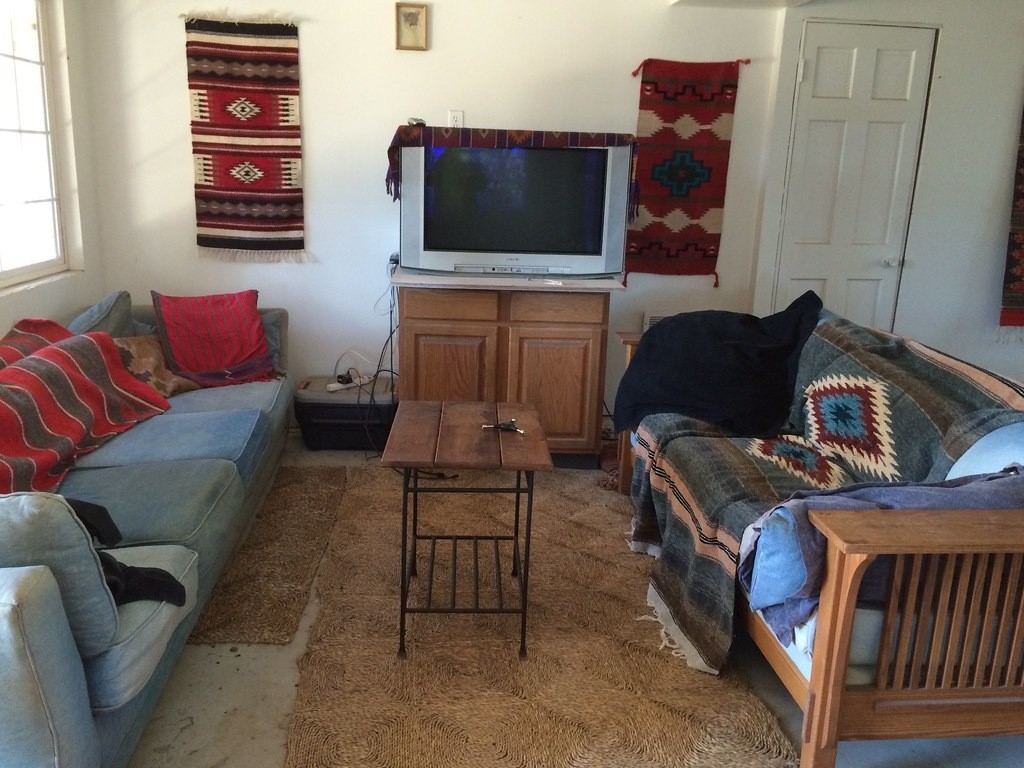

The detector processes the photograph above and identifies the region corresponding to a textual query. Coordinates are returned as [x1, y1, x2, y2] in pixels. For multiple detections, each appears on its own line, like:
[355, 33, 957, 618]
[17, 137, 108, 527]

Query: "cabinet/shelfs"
[391, 264, 626, 466]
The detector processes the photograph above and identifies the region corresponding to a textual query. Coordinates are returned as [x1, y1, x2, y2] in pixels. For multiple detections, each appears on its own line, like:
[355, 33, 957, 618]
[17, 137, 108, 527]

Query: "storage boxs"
[294, 374, 399, 453]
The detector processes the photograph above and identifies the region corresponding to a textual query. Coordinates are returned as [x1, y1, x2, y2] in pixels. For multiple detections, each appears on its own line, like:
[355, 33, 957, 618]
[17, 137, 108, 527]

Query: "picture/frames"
[395, 2, 427, 51]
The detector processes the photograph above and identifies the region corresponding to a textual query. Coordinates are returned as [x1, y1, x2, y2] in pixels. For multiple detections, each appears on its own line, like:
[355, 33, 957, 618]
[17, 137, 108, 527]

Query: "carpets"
[184, 465, 348, 646]
[281, 464, 804, 768]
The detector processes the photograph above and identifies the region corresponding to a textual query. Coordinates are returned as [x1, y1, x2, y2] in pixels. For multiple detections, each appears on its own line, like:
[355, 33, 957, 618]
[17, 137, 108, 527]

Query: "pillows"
[109, 335, 202, 397]
[151, 289, 277, 389]
[66, 290, 157, 340]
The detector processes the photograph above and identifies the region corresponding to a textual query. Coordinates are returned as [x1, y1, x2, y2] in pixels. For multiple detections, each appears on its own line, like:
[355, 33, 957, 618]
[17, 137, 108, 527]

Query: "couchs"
[616, 305, 1024, 767]
[0, 299, 289, 767]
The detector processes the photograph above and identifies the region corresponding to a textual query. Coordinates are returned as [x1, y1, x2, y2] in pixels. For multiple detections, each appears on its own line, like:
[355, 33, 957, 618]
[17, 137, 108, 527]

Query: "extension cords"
[326, 375, 374, 391]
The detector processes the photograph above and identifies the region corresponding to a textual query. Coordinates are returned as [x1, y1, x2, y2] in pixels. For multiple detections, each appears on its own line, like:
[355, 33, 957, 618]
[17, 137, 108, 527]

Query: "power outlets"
[447, 109, 465, 128]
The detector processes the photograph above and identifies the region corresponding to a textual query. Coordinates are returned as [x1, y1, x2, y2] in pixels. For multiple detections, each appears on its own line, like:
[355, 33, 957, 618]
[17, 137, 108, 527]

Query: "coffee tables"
[381, 399, 553, 664]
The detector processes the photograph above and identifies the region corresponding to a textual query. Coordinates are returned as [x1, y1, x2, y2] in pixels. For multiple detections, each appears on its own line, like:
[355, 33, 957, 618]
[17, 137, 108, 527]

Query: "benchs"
[0, 491, 119, 659]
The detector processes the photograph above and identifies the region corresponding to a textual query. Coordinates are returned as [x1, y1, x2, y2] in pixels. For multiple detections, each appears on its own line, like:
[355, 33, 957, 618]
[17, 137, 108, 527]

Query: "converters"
[337, 373, 353, 384]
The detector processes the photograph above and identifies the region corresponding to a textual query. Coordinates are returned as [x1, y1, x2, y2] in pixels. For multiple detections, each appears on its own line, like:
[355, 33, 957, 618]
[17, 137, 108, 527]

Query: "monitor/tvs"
[398, 125, 632, 276]
